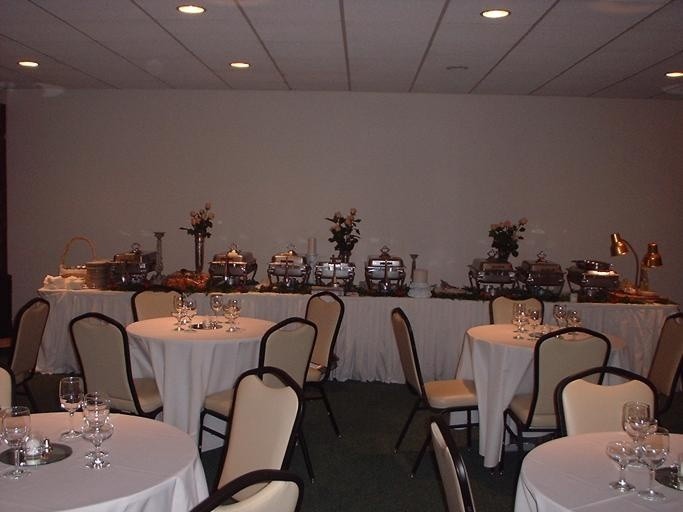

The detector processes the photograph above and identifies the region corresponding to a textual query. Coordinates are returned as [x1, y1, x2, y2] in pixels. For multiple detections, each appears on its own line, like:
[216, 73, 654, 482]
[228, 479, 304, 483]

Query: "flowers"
[324, 207, 361, 257]
[488, 218, 528, 261]
[180, 202, 215, 238]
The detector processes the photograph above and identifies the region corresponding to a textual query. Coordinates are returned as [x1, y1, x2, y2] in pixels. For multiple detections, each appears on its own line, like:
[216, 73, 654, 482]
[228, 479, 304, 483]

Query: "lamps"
[610, 231, 662, 298]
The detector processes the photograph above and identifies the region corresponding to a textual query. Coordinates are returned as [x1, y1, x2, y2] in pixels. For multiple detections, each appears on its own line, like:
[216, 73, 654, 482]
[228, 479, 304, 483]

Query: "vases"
[195, 234, 205, 274]
[500, 251, 508, 261]
[342, 255, 349, 263]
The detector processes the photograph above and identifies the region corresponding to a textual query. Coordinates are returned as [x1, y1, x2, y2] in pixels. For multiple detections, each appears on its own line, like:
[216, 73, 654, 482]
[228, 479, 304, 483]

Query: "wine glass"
[511, 303, 580, 342]
[606, 401, 670, 500]
[1, 378, 117, 481]
[172, 293, 240, 333]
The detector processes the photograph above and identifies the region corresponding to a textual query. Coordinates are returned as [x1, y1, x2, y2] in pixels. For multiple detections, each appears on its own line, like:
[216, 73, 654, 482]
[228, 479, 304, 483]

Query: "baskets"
[58, 236, 97, 284]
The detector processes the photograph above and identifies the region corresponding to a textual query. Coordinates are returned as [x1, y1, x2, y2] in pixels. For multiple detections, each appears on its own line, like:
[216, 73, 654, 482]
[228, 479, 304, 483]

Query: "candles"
[227, 249, 237, 257]
[414, 269, 427, 284]
[309, 237, 316, 254]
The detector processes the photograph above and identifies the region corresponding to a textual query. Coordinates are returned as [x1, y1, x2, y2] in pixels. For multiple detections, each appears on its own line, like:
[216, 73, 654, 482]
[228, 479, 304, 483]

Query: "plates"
[84, 261, 110, 289]
